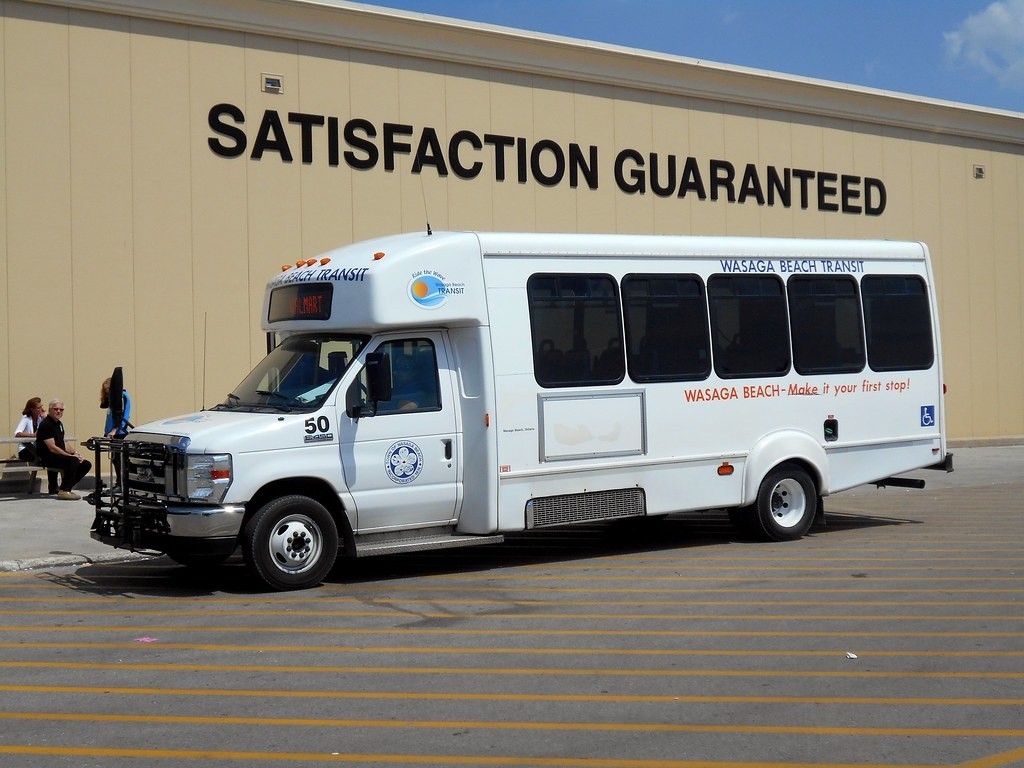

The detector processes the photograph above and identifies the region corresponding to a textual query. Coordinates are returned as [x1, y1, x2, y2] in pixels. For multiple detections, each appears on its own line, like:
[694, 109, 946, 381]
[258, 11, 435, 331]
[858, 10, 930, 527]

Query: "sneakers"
[58, 490, 81, 500]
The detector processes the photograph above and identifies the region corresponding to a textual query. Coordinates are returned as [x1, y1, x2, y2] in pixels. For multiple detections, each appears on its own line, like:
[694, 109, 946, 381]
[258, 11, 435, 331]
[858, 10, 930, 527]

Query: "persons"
[15, 397, 60, 494]
[36, 400, 92, 500]
[100, 377, 131, 493]
[380, 354, 423, 411]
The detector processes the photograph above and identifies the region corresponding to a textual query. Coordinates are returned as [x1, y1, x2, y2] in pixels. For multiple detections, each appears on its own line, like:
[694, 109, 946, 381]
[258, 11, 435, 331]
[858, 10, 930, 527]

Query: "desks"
[0, 437, 78, 495]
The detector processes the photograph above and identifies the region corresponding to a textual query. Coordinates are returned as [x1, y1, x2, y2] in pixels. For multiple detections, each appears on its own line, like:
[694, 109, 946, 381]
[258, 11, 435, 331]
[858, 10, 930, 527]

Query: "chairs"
[723, 332, 765, 375]
[633, 336, 675, 375]
[533, 338, 576, 389]
[593, 338, 632, 383]
[415, 350, 435, 388]
[266, 343, 325, 406]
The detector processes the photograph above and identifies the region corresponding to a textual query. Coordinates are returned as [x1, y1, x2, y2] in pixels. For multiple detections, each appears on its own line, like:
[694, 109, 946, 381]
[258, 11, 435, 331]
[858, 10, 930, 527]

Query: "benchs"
[0, 458, 26, 464]
[0, 466, 62, 473]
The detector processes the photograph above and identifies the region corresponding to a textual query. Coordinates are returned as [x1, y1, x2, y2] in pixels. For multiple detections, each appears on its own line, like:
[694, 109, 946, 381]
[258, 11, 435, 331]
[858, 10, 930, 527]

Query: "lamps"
[972, 164, 985, 179]
[261, 72, 284, 94]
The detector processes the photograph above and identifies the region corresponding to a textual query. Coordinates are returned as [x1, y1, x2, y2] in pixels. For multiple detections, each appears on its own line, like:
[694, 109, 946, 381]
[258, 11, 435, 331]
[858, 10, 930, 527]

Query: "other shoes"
[106, 485, 122, 494]
[49, 493, 59, 497]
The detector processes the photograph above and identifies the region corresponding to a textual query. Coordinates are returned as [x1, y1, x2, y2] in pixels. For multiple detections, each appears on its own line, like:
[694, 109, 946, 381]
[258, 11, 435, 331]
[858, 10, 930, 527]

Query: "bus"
[81, 231, 955, 593]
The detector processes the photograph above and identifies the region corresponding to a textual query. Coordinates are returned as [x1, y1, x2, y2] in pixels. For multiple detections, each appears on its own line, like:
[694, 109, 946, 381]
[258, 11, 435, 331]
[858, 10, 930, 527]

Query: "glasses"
[53, 408, 64, 411]
[396, 364, 414, 372]
